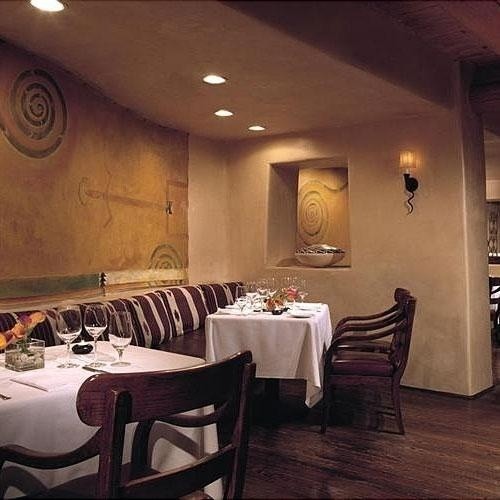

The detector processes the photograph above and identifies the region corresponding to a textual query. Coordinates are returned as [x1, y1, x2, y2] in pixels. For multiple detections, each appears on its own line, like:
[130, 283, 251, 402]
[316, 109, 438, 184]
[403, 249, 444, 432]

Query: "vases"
[4, 336, 46, 371]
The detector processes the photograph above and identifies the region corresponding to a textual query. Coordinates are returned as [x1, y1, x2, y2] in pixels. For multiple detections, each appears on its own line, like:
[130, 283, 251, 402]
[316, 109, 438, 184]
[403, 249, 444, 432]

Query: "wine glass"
[83, 304, 107, 368]
[108, 311, 132, 367]
[56, 304, 82, 369]
[235, 276, 309, 318]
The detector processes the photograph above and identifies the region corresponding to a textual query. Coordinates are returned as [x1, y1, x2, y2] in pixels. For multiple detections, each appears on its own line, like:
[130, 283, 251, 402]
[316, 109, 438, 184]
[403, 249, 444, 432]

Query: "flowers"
[16, 313, 34, 355]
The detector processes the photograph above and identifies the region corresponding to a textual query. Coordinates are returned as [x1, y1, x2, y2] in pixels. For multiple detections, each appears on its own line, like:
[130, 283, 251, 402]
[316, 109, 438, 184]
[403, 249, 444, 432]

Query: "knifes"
[82, 366, 111, 374]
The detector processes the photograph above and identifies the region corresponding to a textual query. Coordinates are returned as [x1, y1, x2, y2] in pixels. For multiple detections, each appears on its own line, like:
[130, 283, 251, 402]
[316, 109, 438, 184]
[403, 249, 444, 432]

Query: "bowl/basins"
[294, 252, 345, 267]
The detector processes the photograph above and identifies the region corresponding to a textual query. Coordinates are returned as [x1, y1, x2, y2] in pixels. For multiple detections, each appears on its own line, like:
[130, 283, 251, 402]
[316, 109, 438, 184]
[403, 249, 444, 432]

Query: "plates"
[220, 305, 250, 315]
[289, 310, 312, 317]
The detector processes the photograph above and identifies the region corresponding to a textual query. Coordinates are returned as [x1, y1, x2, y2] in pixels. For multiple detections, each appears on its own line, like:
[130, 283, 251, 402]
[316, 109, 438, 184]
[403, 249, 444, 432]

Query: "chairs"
[322, 284, 417, 437]
[0, 349, 257, 500]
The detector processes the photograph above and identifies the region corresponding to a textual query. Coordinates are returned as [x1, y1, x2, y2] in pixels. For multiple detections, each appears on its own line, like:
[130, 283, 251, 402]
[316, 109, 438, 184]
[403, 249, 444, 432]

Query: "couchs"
[0, 276, 246, 362]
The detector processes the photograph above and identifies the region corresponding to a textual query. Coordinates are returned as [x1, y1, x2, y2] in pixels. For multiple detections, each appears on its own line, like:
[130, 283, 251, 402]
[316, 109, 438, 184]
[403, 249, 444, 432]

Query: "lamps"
[399, 149, 418, 193]
